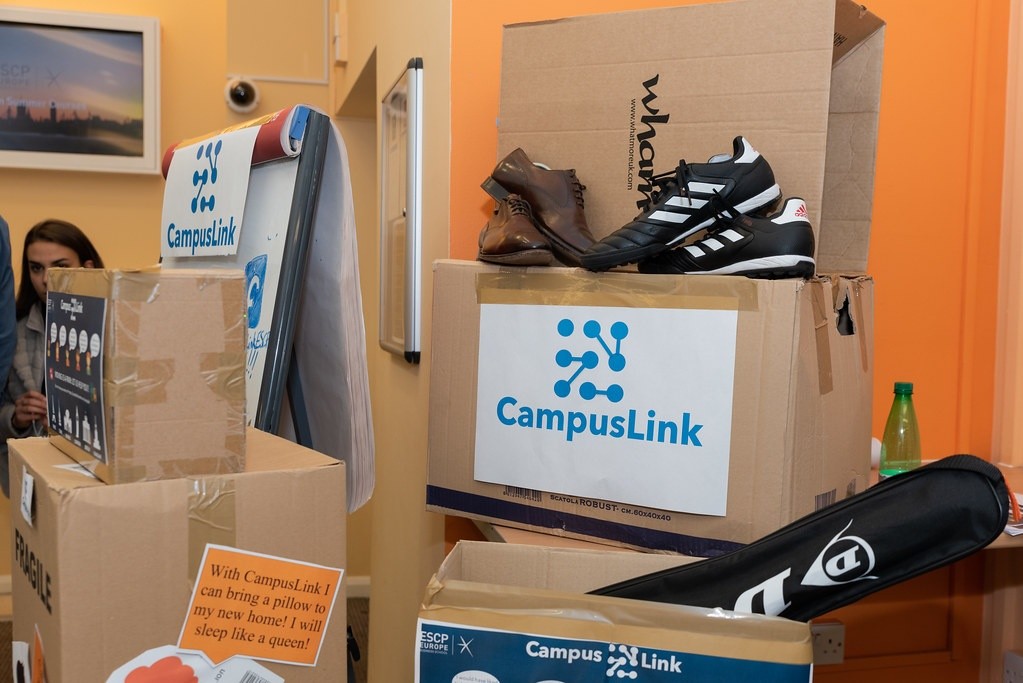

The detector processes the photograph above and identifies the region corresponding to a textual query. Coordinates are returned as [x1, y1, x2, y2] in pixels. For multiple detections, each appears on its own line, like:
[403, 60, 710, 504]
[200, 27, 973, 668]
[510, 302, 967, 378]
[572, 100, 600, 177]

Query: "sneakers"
[579, 135, 783, 269]
[638, 196, 815, 279]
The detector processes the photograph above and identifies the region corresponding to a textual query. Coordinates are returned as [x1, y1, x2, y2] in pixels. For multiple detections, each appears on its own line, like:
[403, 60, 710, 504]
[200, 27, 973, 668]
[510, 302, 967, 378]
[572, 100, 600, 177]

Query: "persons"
[0, 218, 106, 445]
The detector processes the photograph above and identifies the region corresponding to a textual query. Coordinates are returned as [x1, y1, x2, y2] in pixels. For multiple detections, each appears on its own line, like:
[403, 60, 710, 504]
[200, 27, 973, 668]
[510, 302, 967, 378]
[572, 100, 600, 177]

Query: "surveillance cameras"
[225, 78, 259, 113]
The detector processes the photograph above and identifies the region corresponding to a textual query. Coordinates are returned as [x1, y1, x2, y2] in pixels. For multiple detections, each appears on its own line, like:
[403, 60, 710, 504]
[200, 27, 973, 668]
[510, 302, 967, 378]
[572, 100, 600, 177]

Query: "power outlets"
[812, 622, 848, 667]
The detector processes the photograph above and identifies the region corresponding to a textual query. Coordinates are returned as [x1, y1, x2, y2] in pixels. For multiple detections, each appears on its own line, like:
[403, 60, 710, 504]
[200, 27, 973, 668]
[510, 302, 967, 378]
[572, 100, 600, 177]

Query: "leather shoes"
[476, 148, 598, 269]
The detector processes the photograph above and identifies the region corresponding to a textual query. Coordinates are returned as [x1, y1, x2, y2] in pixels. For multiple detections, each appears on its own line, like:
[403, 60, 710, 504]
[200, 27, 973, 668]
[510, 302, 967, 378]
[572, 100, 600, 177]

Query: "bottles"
[878, 380, 921, 482]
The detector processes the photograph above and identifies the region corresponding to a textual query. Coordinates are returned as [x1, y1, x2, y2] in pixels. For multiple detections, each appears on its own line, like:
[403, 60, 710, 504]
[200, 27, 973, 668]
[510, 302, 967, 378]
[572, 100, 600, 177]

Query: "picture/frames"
[0, 6, 165, 176]
[373, 56, 429, 368]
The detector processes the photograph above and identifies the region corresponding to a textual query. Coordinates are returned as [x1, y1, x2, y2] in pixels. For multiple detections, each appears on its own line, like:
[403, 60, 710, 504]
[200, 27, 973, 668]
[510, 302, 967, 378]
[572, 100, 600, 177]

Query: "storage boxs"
[7, 426, 348, 683]
[414, 533, 816, 683]
[497, 1, 890, 279]
[426, 255, 877, 553]
[45, 267, 249, 475]
